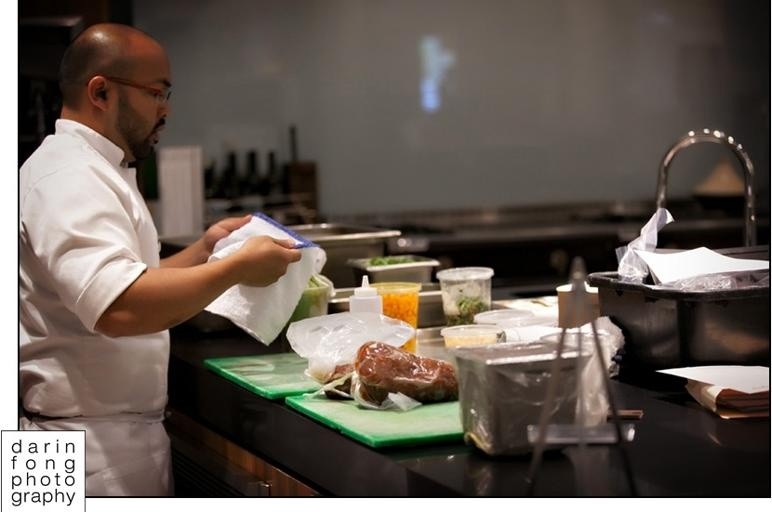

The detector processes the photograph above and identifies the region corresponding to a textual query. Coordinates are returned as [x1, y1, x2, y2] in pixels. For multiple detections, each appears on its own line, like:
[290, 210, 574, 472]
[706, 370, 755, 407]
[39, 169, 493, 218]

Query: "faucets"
[656, 129, 759, 246]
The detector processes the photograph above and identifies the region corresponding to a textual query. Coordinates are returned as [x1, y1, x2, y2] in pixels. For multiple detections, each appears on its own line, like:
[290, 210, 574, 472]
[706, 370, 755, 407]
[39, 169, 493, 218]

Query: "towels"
[198, 214, 329, 347]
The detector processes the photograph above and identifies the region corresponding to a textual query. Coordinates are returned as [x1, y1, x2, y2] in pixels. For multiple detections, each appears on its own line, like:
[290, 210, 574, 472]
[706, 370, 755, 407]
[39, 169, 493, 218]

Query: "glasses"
[86, 74, 170, 103]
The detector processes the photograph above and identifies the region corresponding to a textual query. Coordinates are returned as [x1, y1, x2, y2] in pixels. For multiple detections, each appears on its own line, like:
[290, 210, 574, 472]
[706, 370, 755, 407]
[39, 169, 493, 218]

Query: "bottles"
[349, 275, 383, 320]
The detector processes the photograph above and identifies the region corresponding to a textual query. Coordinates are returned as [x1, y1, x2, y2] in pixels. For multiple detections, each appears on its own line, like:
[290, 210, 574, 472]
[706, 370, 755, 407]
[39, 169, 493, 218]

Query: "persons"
[17, 22, 303, 498]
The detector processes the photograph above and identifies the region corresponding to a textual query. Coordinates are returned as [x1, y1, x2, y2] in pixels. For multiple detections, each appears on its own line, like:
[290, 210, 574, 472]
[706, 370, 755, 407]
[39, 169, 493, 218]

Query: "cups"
[435, 265, 496, 326]
[369, 281, 422, 354]
